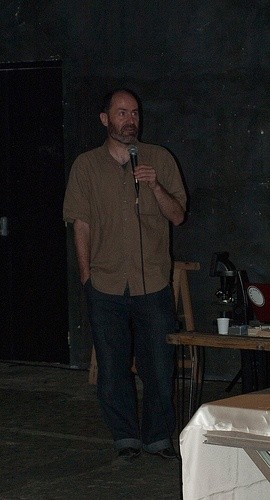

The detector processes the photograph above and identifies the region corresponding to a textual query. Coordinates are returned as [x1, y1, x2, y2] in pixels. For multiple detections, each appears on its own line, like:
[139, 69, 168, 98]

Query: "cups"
[216, 317, 230, 335]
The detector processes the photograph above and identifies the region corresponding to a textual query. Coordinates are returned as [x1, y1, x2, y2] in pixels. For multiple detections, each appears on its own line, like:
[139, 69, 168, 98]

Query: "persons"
[60, 84, 189, 462]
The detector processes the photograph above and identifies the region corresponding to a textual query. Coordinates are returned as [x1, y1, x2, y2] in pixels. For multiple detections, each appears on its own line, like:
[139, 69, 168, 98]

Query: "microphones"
[128, 144, 141, 193]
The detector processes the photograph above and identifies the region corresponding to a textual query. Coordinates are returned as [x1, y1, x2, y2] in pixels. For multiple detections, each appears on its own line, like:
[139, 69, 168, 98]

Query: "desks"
[163, 325, 270, 430]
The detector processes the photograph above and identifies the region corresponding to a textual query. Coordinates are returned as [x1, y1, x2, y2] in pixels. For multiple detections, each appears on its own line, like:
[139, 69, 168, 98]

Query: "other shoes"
[153, 447, 177, 459]
[116, 448, 142, 462]
